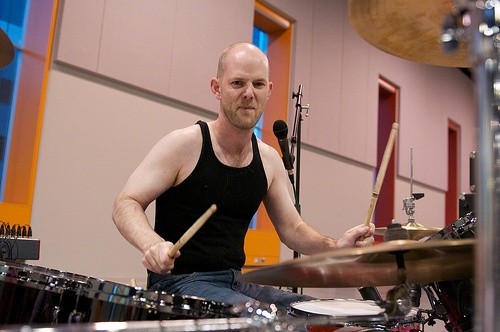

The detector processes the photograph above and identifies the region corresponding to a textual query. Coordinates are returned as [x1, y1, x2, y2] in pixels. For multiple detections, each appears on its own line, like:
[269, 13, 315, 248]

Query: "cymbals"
[237, 239, 477, 287]
[374, 223, 442, 240]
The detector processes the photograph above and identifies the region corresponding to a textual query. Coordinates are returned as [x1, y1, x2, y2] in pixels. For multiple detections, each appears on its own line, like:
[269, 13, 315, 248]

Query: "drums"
[421, 210, 478, 332]
[0, 260, 245, 322]
[287, 297, 389, 332]
[2, 318, 267, 332]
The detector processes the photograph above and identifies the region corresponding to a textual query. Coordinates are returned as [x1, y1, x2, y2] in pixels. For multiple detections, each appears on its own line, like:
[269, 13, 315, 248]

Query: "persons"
[110, 41, 376, 304]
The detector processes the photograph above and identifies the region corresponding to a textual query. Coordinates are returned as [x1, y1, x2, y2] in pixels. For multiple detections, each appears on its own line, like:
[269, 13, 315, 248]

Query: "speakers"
[458, 194, 474, 218]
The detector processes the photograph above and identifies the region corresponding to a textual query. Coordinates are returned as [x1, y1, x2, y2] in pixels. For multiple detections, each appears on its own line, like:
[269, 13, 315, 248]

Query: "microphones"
[272, 119, 295, 185]
